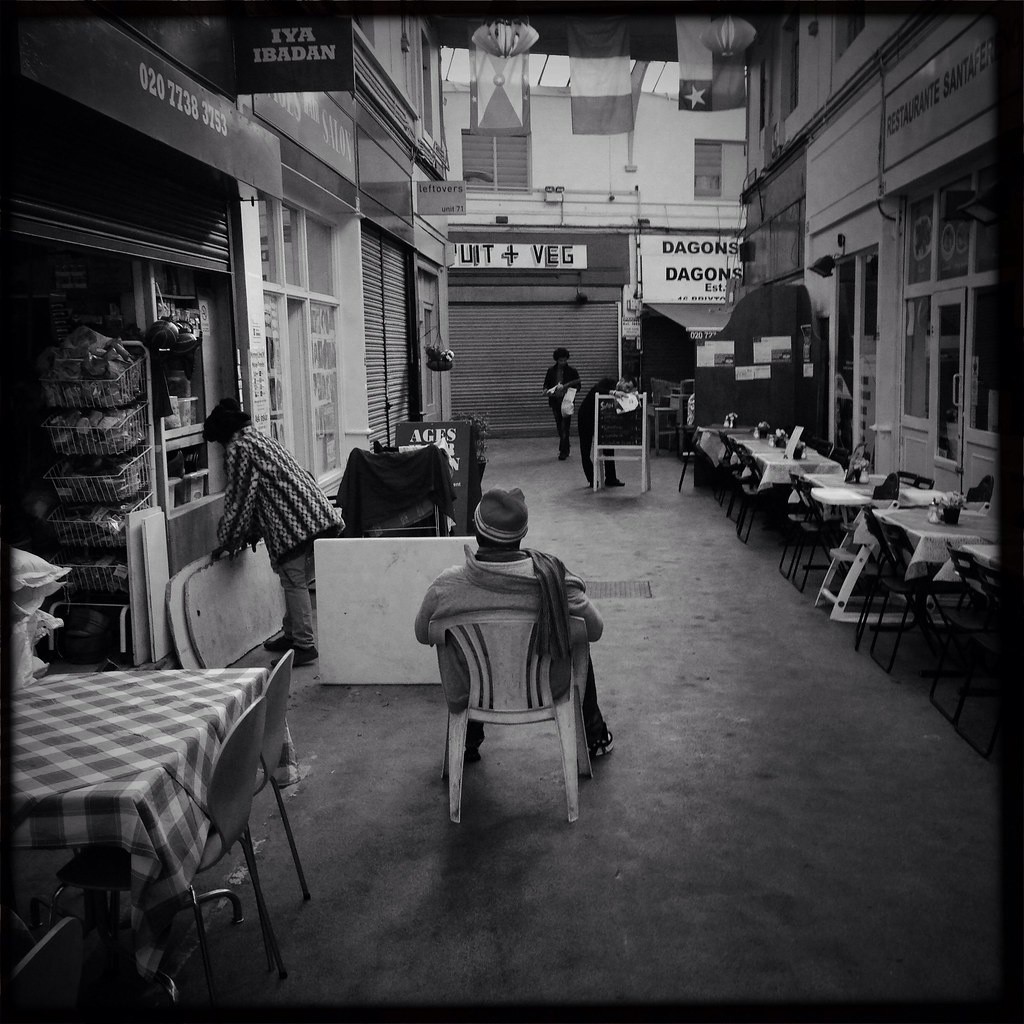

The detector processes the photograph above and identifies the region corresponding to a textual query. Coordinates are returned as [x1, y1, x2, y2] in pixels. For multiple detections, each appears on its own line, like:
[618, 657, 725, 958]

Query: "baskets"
[62, 633, 111, 664]
[40, 356, 150, 594]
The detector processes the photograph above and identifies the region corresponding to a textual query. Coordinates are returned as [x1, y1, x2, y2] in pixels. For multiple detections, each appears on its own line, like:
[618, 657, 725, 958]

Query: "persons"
[543, 347, 581, 461]
[202, 398, 345, 668]
[413, 486, 614, 762]
[578, 375, 639, 488]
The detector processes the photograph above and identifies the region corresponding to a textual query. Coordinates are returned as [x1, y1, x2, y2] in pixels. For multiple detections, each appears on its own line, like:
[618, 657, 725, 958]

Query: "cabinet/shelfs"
[146, 264, 228, 575]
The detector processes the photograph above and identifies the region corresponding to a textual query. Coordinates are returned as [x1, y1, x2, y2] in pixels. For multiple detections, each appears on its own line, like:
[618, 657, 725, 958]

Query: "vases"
[776, 438, 784, 447]
[860, 471, 870, 484]
[729, 420, 736, 428]
[793, 448, 803, 460]
[426, 359, 453, 371]
[943, 508, 961, 525]
[760, 430, 768, 439]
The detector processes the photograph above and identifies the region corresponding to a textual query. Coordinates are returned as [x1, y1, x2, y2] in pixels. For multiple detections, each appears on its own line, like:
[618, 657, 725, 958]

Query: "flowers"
[758, 421, 770, 428]
[853, 459, 869, 471]
[796, 439, 802, 448]
[726, 413, 737, 419]
[935, 490, 968, 512]
[423, 344, 455, 361]
[775, 429, 789, 437]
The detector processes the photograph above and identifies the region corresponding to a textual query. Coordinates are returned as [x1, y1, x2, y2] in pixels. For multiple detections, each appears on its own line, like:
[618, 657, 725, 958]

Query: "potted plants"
[451, 412, 490, 536]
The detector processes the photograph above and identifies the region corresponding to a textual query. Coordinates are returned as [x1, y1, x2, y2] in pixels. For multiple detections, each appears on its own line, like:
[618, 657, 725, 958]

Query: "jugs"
[148, 316, 198, 357]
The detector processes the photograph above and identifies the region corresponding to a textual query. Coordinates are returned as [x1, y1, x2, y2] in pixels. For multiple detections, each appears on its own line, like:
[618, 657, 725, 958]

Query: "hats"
[474, 488, 528, 543]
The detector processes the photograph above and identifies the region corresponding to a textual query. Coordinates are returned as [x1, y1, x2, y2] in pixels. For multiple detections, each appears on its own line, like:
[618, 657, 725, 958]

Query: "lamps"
[808, 254, 835, 278]
[496, 216, 508, 223]
[639, 218, 650, 228]
[545, 185, 565, 203]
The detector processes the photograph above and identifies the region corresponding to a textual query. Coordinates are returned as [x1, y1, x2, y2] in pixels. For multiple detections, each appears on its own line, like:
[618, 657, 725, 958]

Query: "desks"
[933, 545, 998, 580]
[726, 433, 790, 443]
[872, 507, 998, 583]
[693, 425, 754, 440]
[14, 667, 269, 1002]
[751, 452, 845, 492]
[742, 443, 816, 451]
[789, 473, 916, 520]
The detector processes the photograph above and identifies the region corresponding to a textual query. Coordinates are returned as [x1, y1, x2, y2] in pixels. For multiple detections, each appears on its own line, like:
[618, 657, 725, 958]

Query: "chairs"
[427, 612, 588, 825]
[196, 645, 310, 976]
[679, 421, 1000, 724]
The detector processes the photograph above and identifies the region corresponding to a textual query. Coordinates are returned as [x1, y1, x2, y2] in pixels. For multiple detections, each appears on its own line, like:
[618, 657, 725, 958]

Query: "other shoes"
[264, 634, 296, 651]
[589, 730, 614, 756]
[605, 478, 625, 487]
[271, 645, 319, 667]
[464, 750, 481, 763]
[590, 480, 599, 488]
[559, 452, 569, 460]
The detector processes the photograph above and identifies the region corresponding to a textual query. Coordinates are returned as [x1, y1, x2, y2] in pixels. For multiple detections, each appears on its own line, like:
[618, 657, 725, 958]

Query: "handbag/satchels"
[561, 388, 577, 417]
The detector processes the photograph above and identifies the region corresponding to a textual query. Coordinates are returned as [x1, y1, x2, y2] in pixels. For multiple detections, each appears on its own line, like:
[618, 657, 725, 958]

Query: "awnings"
[648, 302, 738, 340]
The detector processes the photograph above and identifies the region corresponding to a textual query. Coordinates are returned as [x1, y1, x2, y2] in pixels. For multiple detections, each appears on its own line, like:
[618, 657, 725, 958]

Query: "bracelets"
[613, 390, 616, 395]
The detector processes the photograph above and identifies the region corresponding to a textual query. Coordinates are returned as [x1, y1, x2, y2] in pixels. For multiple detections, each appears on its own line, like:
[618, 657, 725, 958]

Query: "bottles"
[168, 371, 192, 398]
